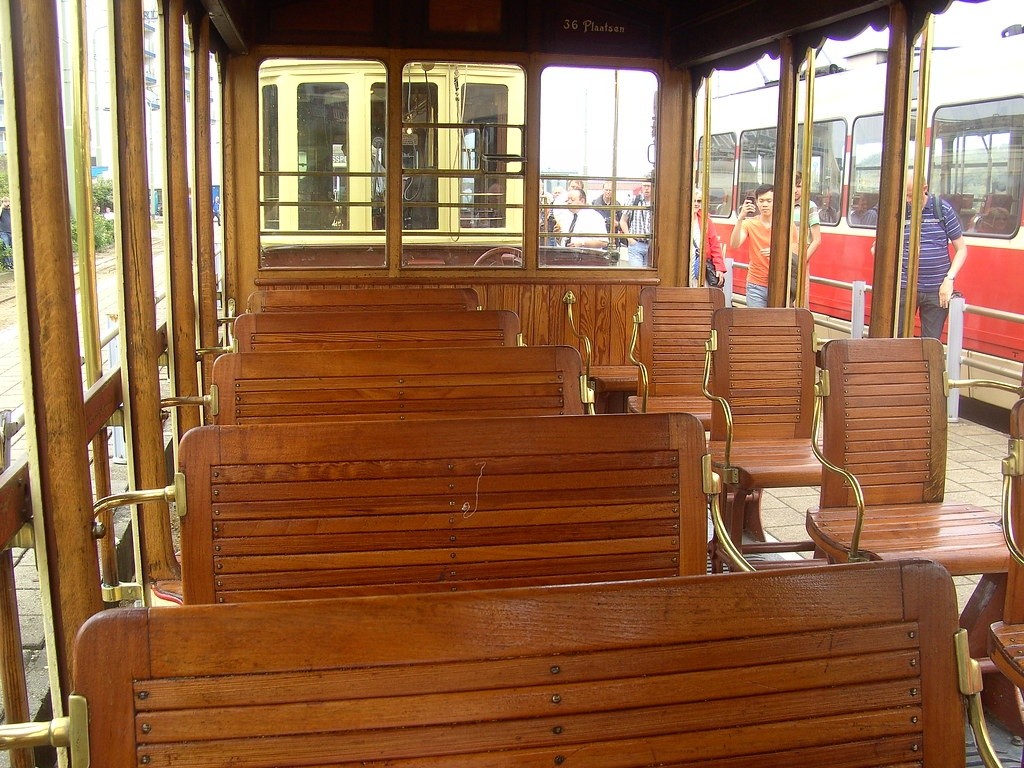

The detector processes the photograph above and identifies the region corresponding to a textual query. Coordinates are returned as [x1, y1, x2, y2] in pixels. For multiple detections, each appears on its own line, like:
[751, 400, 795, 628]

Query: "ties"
[565, 213, 577, 247]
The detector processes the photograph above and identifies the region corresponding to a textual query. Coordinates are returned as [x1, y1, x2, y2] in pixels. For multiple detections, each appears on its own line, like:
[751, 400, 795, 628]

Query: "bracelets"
[945, 274, 954, 280]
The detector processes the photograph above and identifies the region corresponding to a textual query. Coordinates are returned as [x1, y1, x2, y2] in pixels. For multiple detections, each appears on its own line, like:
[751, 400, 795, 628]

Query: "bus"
[239, 41, 527, 269]
[651, 42, 1024, 415]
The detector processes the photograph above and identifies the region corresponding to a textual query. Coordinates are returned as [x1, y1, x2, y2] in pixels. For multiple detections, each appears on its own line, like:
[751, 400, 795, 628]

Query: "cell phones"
[746, 196, 755, 214]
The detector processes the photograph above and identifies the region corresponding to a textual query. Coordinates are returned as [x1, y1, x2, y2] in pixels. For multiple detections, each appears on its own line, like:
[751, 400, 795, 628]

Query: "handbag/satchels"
[620, 222, 630, 247]
[704, 258, 725, 287]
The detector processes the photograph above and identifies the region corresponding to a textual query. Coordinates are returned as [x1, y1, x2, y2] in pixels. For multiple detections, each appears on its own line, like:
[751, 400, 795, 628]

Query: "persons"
[299, 160, 335, 230]
[552, 189, 609, 249]
[0, 196, 14, 272]
[213, 196, 221, 226]
[540, 179, 565, 246]
[790, 171, 821, 307]
[813, 184, 879, 226]
[871, 168, 967, 340]
[567, 180, 584, 192]
[730, 183, 798, 307]
[620, 181, 652, 267]
[692, 187, 728, 292]
[188, 187, 194, 267]
[624, 193, 631, 206]
[592, 182, 622, 250]
[977, 207, 1010, 234]
[468, 183, 504, 227]
[342, 141, 386, 214]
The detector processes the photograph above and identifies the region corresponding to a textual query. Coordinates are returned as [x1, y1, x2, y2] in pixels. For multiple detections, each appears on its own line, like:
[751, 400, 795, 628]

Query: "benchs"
[244, 288, 482, 314]
[68, 556, 1003, 768]
[173, 413, 756, 606]
[207, 346, 594, 425]
[233, 310, 527, 354]
[252, 278, 662, 414]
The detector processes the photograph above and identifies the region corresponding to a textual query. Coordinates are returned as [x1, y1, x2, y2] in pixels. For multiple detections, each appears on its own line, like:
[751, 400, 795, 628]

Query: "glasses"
[693, 199, 702, 203]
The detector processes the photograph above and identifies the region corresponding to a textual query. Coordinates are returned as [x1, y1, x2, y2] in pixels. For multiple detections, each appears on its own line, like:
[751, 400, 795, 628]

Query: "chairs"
[985, 398, 1024, 768]
[700, 307, 824, 574]
[806, 337, 1024, 741]
[625, 286, 726, 431]
